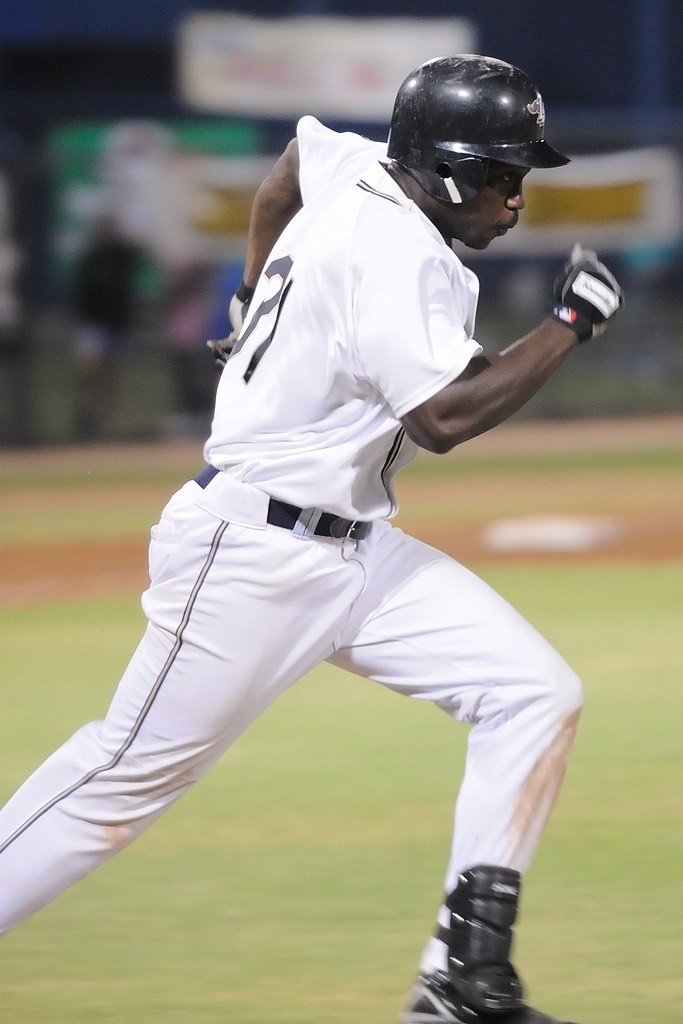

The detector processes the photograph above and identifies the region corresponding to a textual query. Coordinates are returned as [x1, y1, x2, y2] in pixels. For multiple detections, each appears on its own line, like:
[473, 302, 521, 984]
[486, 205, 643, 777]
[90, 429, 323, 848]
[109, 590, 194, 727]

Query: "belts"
[193, 464, 374, 544]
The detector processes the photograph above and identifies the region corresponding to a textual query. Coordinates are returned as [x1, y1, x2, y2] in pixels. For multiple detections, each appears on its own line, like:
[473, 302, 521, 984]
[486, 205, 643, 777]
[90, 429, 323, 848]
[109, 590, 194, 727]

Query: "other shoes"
[407, 966, 572, 1024]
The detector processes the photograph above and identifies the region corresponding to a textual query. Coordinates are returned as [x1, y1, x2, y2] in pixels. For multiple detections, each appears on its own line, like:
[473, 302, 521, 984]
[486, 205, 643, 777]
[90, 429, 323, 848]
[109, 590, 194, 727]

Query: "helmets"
[381, 52, 571, 209]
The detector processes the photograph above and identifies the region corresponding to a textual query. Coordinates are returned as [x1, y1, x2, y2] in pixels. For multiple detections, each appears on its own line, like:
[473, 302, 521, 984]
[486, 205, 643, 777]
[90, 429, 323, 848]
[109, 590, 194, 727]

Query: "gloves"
[206, 280, 255, 365]
[547, 236, 624, 344]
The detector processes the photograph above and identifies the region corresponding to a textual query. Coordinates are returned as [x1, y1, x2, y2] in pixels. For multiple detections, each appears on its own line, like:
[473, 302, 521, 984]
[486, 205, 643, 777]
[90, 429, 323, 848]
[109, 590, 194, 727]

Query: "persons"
[0, 54, 625, 1024]
